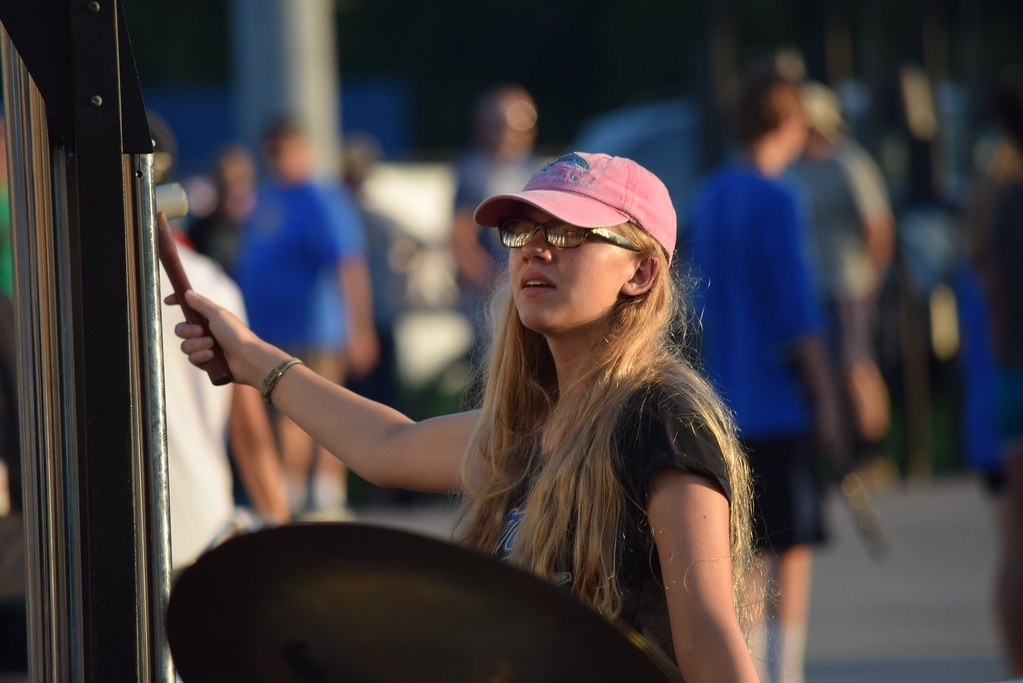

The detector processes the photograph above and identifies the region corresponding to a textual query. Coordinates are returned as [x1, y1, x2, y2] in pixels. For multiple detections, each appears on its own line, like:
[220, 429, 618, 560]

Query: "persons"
[164, 152, 760, 683]
[146, 72, 1023, 683]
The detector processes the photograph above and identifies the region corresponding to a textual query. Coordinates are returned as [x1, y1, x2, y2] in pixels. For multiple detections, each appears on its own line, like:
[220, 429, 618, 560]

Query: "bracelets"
[261, 357, 304, 404]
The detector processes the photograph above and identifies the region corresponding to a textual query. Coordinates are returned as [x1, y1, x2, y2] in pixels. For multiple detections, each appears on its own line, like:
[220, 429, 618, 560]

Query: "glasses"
[497, 216, 643, 252]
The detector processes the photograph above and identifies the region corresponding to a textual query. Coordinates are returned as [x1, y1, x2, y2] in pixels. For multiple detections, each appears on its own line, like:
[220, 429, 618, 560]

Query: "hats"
[474, 152, 677, 270]
